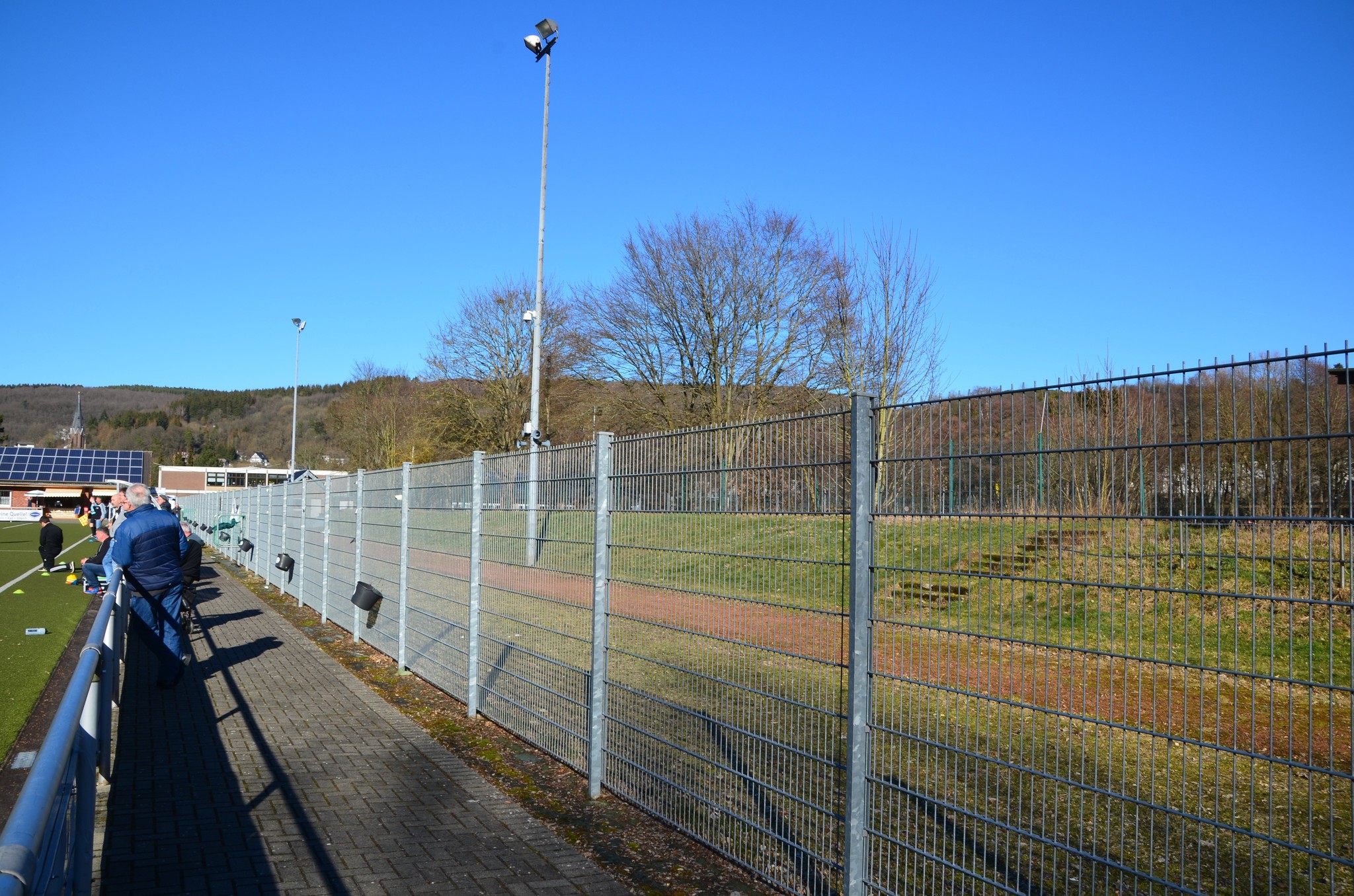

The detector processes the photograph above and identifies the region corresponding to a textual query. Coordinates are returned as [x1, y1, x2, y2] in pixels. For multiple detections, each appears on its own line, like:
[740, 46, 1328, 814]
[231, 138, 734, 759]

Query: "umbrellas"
[24, 490, 45, 507]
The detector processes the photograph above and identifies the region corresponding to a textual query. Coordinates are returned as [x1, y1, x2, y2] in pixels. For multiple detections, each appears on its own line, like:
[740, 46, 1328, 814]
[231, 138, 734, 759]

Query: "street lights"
[290, 317, 308, 482]
[522, 17, 559, 568]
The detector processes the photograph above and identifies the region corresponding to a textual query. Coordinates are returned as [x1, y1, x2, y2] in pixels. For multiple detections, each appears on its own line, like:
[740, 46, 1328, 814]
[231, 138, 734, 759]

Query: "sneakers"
[38, 567, 48, 572]
[84, 587, 102, 594]
[69, 561, 75, 573]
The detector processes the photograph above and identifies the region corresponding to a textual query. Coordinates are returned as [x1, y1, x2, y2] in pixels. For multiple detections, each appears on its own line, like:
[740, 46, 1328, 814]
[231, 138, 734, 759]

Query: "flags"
[78, 513, 88, 526]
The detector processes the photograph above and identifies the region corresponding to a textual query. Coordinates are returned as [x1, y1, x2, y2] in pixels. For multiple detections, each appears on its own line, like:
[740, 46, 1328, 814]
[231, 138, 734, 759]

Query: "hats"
[160, 495, 167, 501]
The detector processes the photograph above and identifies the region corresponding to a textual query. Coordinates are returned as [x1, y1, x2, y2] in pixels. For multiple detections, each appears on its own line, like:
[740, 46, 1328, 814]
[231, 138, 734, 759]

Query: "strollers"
[180, 539, 201, 635]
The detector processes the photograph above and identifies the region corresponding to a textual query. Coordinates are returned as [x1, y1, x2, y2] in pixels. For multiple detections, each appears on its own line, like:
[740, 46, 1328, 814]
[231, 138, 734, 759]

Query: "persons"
[157, 495, 172, 514]
[25, 496, 32, 510]
[111, 482, 192, 691]
[98, 487, 129, 598]
[122, 498, 131, 512]
[172, 503, 183, 521]
[88, 496, 98, 538]
[38, 516, 75, 573]
[92, 496, 106, 542]
[81, 526, 113, 594]
[55, 500, 62, 508]
[182, 521, 204, 590]
[108, 494, 122, 529]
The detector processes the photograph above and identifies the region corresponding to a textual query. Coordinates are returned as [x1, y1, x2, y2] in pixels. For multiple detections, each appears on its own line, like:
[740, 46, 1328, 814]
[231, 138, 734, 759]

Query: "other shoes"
[178, 653, 191, 679]
[152, 677, 173, 689]
[89, 536, 95, 538]
[96, 539, 99, 542]
[94, 535, 96, 537]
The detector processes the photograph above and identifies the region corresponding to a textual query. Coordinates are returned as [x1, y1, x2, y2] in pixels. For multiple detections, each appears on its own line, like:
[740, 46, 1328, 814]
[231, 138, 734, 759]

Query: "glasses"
[123, 501, 127, 504]
[126, 498, 128, 501]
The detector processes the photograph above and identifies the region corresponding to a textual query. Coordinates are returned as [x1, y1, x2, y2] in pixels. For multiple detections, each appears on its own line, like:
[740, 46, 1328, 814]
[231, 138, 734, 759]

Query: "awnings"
[44, 488, 83, 497]
[92, 488, 117, 496]
